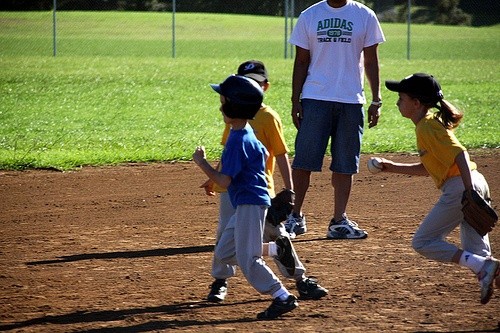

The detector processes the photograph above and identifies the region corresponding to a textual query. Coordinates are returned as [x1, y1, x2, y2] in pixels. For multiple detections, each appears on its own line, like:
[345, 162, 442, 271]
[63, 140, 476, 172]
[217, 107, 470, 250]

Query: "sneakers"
[297, 275, 328, 298]
[275, 236, 295, 276]
[494, 261, 500, 287]
[478, 256, 500, 304]
[257, 295, 299, 319]
[327, 213, 368, 239]
[207, 278, 228, 300]
[284, 214, 307, 239]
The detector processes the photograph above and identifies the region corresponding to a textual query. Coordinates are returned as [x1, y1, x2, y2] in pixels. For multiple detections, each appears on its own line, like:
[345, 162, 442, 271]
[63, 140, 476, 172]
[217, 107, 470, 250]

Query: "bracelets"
[371, 100, 383, 107]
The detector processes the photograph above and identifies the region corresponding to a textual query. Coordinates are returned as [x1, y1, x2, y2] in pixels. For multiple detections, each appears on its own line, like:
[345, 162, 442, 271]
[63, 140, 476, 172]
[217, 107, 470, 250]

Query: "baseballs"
[368, 157, 384, 173]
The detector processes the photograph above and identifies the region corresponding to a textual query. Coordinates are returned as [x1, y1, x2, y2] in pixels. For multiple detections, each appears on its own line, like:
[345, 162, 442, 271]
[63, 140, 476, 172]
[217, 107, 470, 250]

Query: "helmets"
[211, 75, 263, 119]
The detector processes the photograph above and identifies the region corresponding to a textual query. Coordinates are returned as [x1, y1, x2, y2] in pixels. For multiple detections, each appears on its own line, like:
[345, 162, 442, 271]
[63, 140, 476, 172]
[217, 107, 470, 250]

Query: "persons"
[284, 0, 385, 238]
[379, 73, 500, 304]
[191, 74, 299, 321]
[207, 61, 329, 305]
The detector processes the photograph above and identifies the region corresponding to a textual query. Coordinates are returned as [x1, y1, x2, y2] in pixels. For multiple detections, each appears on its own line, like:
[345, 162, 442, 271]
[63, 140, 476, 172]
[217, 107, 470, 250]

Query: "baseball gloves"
[267, 189, 295, 226]
[460, 189, 497, 239]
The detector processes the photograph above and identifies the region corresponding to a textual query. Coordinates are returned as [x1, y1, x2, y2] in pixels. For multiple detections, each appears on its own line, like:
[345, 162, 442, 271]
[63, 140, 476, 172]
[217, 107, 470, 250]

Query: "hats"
[385, 73, 442, 109]
[237, 60, 269, 83]
